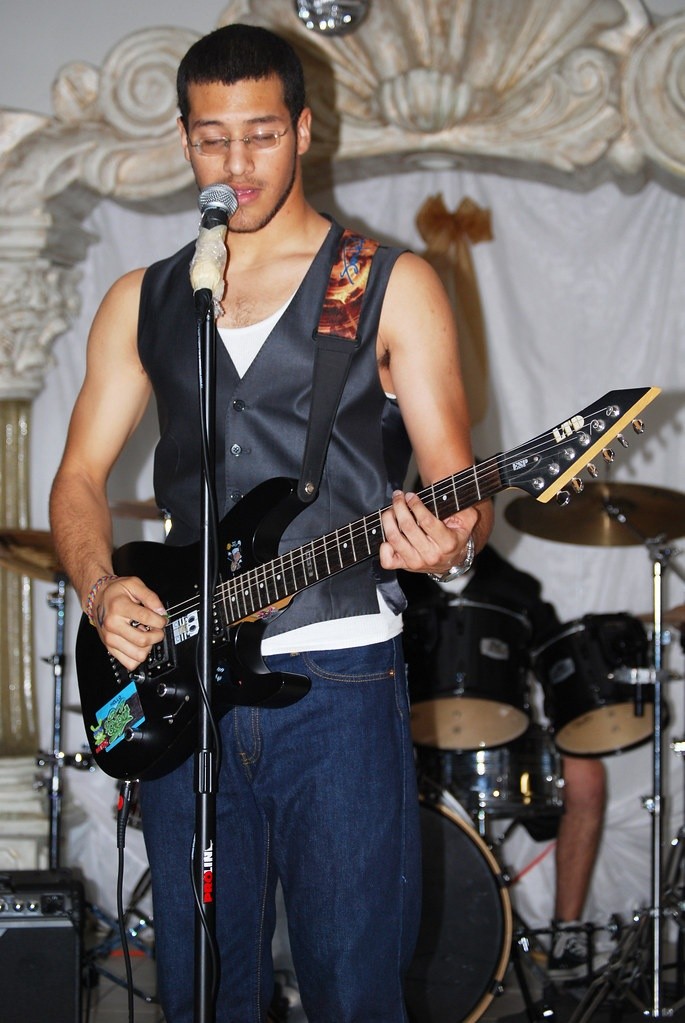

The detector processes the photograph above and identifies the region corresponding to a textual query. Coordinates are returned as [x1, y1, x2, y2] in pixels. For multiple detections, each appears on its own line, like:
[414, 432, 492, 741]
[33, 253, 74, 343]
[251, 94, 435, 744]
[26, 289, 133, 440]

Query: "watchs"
[428, 538, 475, 583]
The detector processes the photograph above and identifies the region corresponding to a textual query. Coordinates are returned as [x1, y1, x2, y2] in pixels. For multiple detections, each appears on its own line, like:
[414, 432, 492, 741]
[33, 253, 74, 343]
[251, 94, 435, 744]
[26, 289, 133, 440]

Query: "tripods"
[567, 495, 685, 1023]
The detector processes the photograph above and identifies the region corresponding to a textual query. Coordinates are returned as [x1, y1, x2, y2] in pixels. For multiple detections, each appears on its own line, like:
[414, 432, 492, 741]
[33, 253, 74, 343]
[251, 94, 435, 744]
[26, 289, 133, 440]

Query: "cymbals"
[0, 528, 72, 587]
[638, 604, 685, 630]
[505, 481, 685, 547]
[111, 498, 164, 521]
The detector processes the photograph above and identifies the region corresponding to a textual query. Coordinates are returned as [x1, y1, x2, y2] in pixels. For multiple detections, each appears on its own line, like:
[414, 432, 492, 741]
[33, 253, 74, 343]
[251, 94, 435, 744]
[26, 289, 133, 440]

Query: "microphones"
[189, 184, 239, 320]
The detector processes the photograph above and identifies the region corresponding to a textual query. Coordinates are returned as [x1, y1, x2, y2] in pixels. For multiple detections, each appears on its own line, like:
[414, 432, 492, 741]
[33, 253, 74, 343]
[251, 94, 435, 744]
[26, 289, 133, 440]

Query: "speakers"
[0, 866, 88, 1023]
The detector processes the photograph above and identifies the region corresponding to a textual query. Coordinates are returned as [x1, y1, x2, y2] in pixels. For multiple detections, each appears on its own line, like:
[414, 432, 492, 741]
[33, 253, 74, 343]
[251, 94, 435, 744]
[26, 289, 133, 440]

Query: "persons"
[48, 23, 494, 1023]
[393, 458, 604, 983]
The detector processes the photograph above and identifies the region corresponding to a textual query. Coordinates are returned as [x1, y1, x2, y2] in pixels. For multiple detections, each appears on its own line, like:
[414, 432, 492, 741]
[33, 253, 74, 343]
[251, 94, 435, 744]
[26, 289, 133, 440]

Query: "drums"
[530, 613, 671, 759]
[418, 719, 566, 817]
[402, 597, 536, 751]
[403, 774, 512, 1023]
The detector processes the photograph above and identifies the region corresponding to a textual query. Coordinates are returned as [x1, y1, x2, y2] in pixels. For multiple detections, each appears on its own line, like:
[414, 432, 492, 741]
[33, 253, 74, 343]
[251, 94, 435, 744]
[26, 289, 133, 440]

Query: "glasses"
[184, 104, 305, 157]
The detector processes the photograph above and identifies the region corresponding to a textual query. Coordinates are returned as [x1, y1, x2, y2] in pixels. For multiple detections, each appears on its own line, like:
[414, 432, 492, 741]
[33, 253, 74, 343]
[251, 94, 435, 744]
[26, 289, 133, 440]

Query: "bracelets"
[87, 575, 117, 624]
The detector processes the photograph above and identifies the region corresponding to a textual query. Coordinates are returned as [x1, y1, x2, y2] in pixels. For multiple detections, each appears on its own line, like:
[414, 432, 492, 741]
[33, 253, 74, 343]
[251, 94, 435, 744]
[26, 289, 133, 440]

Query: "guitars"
[75, 386, 664, 782]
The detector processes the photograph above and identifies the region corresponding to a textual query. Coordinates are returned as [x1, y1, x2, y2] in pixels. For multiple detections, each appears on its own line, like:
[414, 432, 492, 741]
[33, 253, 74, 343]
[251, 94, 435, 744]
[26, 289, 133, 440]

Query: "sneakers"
[544, 913, 613, 984]
